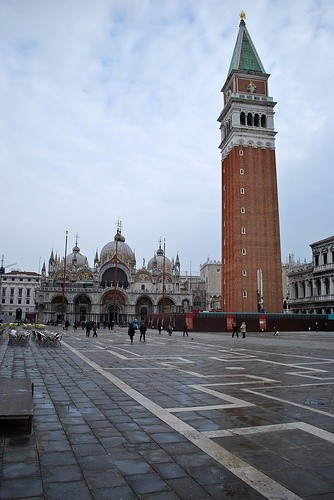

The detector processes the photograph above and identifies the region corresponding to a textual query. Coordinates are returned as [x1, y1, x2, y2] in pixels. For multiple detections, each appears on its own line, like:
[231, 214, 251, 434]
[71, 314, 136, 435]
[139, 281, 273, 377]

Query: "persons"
[232, 320, 239, 338]
[108, 322, 110, 330]
[110, 322, 113, 330]
[167, 322, 173, 336]
[240, 322, 246, 338]
[139, 323, 147, 342]
[85, 323, 91, 337]
[65, 320, 70, 329]
[82, 321, 85, 330]
[158, 321, 162, 334]
[128, 323, 136, 344]
[48, 320, 50, 326]
[103, 321, 107, 329]
[92, 322, 98, 337]
[315, 322, 319, 332]
[274, 324, 279, 336]
[182, 322, 189, 337]
[62, 321, 65, 329]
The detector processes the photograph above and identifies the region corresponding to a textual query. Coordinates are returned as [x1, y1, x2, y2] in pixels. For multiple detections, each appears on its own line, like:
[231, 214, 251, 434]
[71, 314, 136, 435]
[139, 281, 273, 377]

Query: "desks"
[17, 330, 27, 347]
[41, 330, 56, 347]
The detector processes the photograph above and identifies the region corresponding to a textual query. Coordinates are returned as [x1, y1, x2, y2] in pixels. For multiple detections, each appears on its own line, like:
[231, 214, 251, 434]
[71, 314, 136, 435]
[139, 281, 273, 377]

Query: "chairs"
[8, 329, 62, 347]
